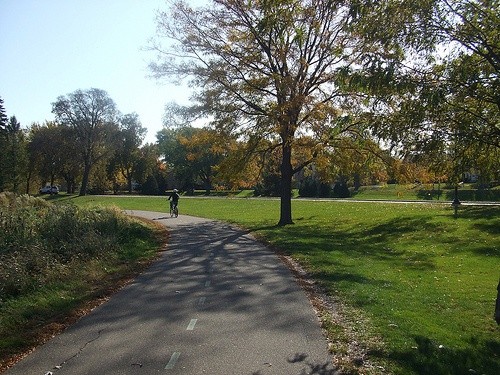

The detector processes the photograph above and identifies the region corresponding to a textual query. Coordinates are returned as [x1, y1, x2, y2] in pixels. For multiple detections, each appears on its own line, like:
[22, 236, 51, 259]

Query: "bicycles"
[168, 199, 179, 218]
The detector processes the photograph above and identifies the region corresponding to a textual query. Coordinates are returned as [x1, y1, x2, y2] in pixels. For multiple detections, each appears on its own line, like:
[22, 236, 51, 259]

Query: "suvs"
[39, 185, 59, 195]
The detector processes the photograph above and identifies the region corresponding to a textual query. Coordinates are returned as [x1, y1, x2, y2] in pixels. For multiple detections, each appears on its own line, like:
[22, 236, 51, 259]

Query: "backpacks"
[173, 194, 179, 201]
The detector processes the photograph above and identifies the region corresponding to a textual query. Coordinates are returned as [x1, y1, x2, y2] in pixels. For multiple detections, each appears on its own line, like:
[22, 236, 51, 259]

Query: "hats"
[174, 189, 178, 192]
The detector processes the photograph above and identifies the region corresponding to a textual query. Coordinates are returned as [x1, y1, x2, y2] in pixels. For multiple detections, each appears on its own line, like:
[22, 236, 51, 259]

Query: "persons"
[168, 189, 179, 215]
